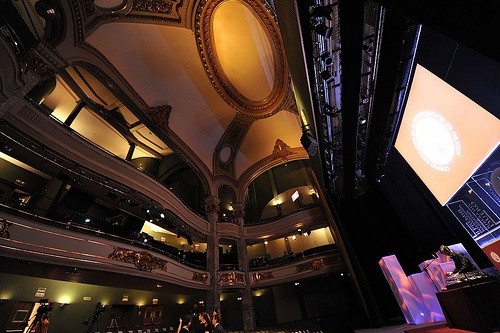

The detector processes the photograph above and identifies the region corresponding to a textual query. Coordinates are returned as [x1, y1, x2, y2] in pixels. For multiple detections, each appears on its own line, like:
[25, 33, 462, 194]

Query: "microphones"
[432, 254, 438, 260]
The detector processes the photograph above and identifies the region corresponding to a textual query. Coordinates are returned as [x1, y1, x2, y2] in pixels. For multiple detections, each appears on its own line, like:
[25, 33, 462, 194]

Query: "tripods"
[87, 315, 99, 333]
[26, 313, 42, 333]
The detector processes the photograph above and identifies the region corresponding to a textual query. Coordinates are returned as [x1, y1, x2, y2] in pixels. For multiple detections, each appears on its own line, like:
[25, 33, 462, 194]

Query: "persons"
[87, 302, 108, 332]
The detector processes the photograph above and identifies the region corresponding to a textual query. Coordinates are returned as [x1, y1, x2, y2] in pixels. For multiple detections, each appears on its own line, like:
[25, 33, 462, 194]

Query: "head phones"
[440, 246, 450, 253]
